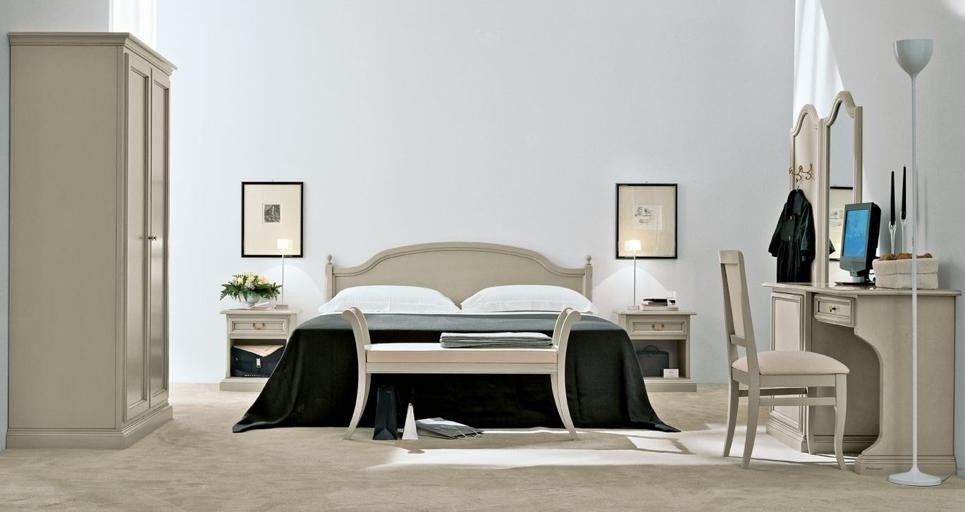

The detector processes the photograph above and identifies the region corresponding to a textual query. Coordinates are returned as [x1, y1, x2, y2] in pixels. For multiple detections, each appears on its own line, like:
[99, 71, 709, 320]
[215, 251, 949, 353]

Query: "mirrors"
[820, 89, 862, 286]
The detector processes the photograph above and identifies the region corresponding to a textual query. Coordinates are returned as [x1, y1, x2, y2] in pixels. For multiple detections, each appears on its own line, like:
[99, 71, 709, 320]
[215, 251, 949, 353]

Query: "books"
[640, 300, 678, 310]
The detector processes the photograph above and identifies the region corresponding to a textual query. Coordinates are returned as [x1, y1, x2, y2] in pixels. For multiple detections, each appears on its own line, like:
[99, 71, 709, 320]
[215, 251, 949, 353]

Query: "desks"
[759, 281, 961, 477]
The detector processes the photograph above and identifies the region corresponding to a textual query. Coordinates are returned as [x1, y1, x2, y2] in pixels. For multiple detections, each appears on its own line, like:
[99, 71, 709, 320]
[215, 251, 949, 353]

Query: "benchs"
[337, 303, 586, 439]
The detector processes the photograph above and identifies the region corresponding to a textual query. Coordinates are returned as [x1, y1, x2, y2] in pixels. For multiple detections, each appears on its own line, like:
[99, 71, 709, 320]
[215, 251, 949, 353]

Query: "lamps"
[622, 237, 642, 310]
[895, 37, 940, 488]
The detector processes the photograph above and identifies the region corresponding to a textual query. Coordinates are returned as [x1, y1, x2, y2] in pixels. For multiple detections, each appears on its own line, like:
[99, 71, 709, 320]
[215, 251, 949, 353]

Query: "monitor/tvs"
[834, 202, 881, 287]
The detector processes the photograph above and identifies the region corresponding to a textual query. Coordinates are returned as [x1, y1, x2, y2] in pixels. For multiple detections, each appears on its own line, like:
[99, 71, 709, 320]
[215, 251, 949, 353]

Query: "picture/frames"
[240, 181, 305, 257]
[826, 183, 856, 263]
[616, 182, 678, 259]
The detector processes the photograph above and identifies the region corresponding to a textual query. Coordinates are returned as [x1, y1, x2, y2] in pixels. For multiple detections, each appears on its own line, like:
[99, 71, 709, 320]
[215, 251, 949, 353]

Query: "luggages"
[231, 344, 285, 376]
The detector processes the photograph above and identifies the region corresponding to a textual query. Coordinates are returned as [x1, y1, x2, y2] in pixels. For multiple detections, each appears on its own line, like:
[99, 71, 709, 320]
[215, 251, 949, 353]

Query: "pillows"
[463, 283, 600, 314]
[316, 283, 461, 316]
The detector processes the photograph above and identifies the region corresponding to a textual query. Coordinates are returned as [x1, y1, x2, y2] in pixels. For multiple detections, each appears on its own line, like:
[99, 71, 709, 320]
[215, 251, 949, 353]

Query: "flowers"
[215, 271, 282, 303]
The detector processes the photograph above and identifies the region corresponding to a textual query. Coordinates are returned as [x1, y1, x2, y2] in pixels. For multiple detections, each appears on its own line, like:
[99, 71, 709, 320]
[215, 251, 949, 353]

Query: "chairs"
[711, 249, 852, 474]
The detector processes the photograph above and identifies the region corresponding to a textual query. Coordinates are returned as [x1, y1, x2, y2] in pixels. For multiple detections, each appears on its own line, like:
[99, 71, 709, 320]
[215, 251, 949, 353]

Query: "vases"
[239, 295, 259, 308]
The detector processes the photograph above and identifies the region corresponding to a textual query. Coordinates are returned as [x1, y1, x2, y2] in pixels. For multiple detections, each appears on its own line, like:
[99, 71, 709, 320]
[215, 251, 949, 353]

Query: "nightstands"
[612, 308, 697, 392]
[219, 308, 299, 394]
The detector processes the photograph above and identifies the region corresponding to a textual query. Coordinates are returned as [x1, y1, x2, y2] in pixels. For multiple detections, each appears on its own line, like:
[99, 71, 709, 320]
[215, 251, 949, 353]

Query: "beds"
[228, 239, 682, 434]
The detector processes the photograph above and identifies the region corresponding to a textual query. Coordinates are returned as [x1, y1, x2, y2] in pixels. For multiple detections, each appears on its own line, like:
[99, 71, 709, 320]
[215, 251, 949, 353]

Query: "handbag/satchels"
[636, 344, 670, 377]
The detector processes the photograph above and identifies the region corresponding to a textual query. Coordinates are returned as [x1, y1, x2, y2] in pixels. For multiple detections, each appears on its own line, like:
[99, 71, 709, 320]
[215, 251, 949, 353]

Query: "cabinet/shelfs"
[8, 29, 178, 453]
